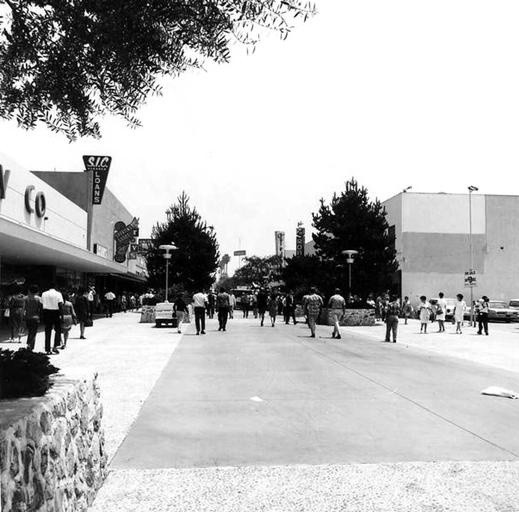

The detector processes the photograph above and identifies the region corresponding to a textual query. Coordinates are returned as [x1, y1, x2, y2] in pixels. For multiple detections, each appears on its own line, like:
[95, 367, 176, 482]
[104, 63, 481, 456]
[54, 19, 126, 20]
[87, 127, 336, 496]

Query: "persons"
[348, 289, 489, 342]
[172, 287, 346, 339]
[0, 283, 156, 354]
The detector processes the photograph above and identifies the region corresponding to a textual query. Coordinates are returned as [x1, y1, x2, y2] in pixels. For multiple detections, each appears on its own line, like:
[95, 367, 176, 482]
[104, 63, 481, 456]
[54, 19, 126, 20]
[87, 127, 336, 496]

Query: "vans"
[202, 286, 263, 309]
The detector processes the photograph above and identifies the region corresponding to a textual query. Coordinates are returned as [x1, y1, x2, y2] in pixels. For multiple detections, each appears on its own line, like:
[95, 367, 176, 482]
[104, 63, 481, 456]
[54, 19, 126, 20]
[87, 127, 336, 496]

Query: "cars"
[509, 299, 519, 310]
[477, 299, 519, 322]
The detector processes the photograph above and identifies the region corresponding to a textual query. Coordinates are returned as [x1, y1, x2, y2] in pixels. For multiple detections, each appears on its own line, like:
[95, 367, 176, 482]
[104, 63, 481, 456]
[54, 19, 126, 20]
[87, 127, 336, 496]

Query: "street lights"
[341, 249, 360, 298]
[159, 243, 181, 299]
[467, 185, 481, 327]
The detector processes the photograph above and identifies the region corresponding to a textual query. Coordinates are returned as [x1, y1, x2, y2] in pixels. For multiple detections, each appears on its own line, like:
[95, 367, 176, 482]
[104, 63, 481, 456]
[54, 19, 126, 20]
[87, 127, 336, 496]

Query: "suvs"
[414, 298, 458, 319]
